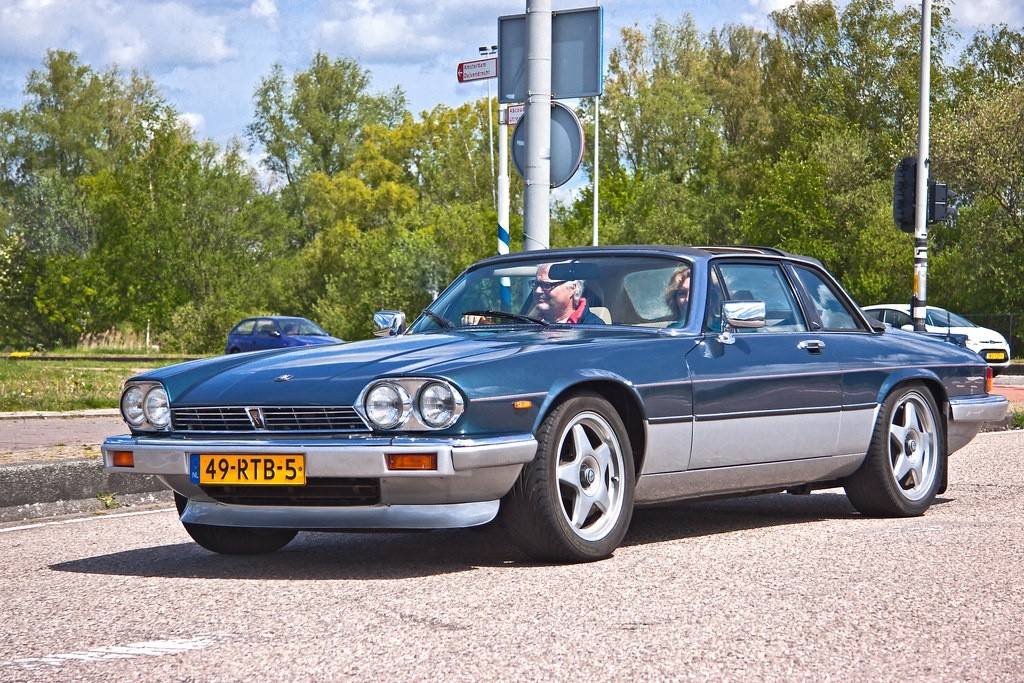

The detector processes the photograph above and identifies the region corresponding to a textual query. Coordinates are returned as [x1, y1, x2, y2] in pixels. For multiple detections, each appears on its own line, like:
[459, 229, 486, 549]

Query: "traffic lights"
[932, 183, 958, 221]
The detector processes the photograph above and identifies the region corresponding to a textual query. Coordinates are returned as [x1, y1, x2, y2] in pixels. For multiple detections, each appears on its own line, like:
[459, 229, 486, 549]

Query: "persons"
[664, 265, 757, 335]
[287, 323, 301, 334]
[461, 259, 608, 326]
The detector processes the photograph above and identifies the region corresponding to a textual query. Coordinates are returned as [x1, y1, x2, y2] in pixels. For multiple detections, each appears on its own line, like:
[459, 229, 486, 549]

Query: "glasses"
[528, 279, 566, 293]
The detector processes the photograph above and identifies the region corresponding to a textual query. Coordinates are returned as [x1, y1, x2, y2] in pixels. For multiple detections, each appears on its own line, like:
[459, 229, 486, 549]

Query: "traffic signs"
[509, 105, 527, 125]
[455, 55, 497, 82]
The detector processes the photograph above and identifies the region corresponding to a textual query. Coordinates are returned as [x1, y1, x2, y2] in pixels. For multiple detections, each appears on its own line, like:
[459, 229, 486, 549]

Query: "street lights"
[478, 45, 498, 208]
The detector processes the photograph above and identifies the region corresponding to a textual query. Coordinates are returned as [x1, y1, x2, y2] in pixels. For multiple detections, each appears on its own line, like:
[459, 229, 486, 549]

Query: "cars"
[96, 234, 1005, 563]
[859, 303, 1011, 376]
[224, 315, 345, 353]
